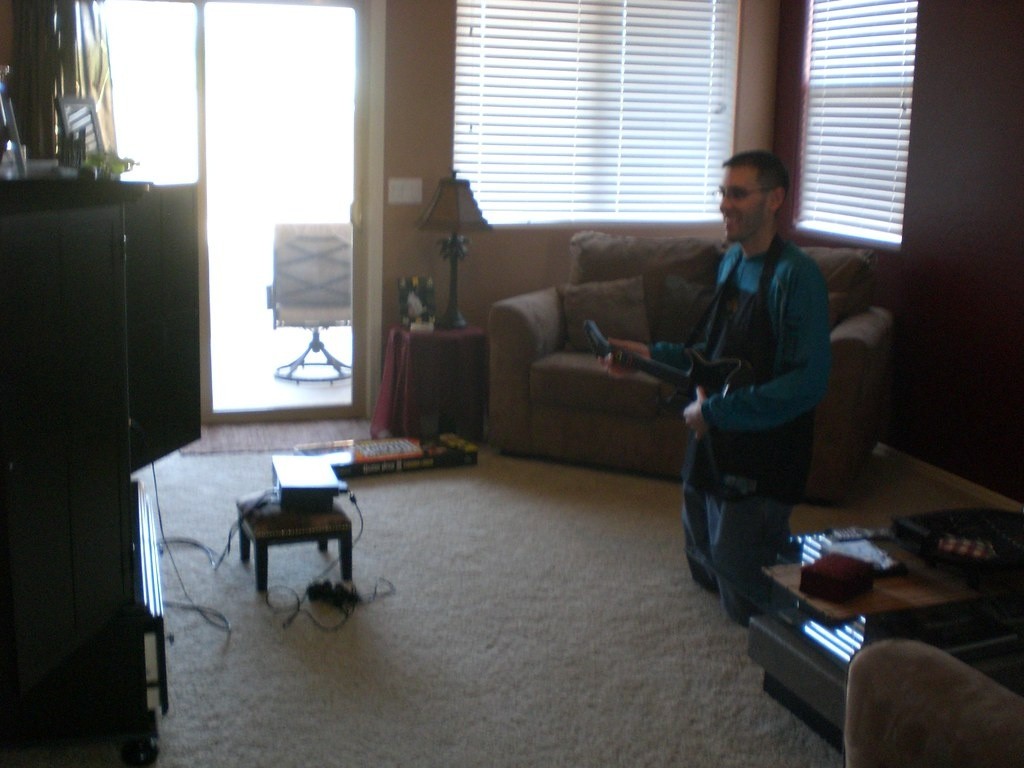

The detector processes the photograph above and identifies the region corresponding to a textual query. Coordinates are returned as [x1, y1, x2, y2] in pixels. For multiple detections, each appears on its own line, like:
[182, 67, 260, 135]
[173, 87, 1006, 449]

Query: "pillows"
[556, 274, 653, 353]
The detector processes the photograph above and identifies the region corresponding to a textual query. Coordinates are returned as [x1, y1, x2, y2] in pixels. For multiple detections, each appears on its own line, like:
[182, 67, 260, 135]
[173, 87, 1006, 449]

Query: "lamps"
[412, 171, 493, 328]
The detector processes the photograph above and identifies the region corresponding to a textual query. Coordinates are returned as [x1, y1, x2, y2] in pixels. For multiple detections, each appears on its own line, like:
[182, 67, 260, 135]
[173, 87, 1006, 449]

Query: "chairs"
[263, 223, 352, 384]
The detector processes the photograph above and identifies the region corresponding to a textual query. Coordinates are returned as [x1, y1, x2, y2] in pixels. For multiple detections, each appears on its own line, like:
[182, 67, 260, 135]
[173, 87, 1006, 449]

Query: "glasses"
[720, 185, 772, 200]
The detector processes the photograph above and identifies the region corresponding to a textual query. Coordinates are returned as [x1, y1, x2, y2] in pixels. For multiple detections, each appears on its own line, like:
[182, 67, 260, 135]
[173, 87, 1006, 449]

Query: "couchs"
[843, 637, 1023, 768]
[492, 228, 900, 500]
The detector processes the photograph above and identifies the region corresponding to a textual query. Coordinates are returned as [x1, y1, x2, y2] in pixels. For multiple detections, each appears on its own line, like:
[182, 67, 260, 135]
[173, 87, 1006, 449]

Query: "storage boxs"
[330, 433, 479, 480]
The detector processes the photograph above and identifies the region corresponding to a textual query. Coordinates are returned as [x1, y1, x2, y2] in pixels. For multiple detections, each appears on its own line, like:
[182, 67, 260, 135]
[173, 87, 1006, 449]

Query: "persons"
[594, 150, 832, 628]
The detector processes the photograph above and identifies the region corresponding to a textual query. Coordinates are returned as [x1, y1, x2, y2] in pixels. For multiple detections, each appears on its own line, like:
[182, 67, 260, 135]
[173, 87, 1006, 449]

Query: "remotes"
[832, 526, 893, 541]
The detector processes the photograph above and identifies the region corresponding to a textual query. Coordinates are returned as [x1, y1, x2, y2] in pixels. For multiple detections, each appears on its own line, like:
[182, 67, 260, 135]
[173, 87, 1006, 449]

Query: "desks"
[369, 323, 486, 442]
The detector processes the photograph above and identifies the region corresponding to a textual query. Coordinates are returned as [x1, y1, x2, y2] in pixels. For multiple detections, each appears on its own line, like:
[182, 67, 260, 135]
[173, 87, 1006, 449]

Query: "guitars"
[582, 319, 747, 414]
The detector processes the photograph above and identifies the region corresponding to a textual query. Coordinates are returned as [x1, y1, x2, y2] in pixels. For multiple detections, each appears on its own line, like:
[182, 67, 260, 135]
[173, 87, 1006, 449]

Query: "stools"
[238, 497, 354, 589]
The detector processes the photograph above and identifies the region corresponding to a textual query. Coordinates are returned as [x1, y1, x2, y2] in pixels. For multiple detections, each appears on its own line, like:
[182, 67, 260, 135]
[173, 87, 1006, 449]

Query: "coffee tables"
[741, 506, 1024, 754]
